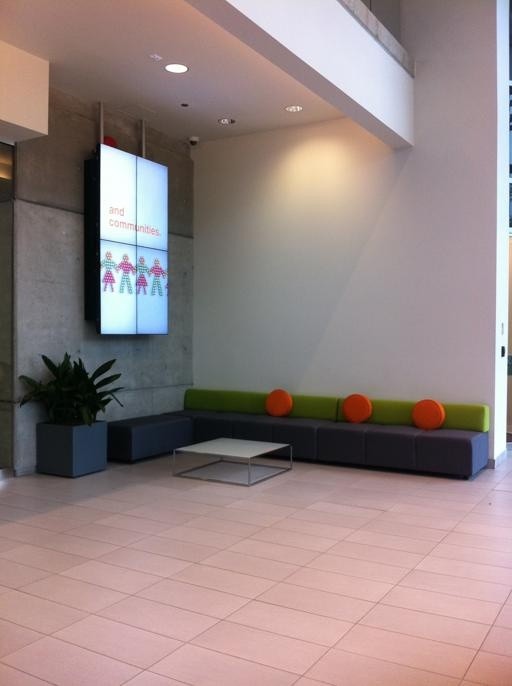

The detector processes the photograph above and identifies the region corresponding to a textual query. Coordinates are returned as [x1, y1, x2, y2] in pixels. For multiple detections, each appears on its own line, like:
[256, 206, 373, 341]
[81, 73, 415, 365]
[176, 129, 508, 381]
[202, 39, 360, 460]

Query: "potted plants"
[18, 352, 126, 478]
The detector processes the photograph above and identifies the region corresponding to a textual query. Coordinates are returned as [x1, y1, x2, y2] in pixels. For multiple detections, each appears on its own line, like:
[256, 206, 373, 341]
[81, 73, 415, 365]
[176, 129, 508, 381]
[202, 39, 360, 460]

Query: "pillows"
[412, 399, 445, 430]
[266, 389, 293, 417]
[343, 394, 373, 423]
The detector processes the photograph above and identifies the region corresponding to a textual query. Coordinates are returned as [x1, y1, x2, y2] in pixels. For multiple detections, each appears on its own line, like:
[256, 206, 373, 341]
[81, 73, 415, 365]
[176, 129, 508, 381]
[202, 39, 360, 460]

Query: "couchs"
[318, 398, 377, 466]
[415, 389, 490, 480]
[365, 399, 427, 470]
[233, 390, 283, 455]
[194, 388, 257, 442]
[161, 389, 217, 444]
[107, 415, 194, 465]
[273, 395, 338, 460]
